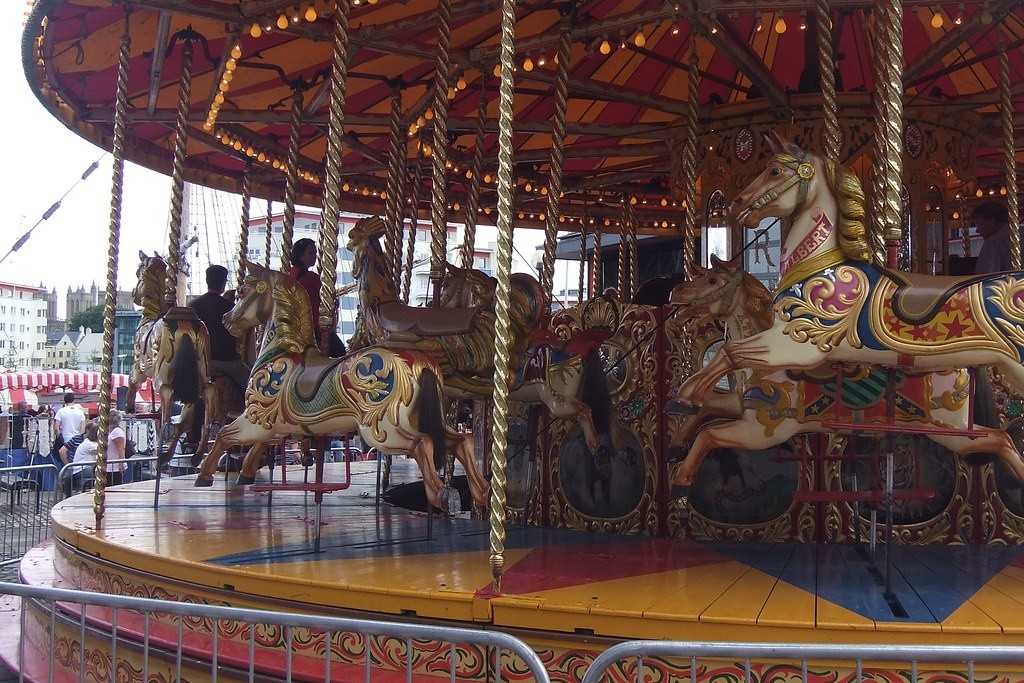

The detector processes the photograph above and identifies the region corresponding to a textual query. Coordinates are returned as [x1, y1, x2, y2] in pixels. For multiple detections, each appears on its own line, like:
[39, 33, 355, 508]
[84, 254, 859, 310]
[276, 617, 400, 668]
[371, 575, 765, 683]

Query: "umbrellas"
[0, 369, 147, 407]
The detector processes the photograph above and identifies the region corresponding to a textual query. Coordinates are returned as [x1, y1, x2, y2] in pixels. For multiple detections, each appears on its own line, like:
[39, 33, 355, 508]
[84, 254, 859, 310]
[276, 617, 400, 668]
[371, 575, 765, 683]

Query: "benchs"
[0, 474, 40, 515]
[139, 470, 169, 481]
[76, 478, 97, 492]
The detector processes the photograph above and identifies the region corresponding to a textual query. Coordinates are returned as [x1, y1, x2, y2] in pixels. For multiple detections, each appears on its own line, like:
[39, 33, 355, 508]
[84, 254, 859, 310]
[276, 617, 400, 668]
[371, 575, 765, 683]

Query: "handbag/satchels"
[125, 439, 136, 459]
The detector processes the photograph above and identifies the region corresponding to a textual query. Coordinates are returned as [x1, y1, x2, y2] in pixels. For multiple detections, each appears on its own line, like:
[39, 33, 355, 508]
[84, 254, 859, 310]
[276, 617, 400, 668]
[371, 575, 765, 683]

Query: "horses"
[124, 250, 219, 467]
[345, 303, 373, 353]
[427, 261, 546, 333]
[167, 325, 258, 467]
[664, 253, 1024, 487]
[346, 214, 612, 469]
[194, 257, 492, 516]
[663, 128, 1024, 417]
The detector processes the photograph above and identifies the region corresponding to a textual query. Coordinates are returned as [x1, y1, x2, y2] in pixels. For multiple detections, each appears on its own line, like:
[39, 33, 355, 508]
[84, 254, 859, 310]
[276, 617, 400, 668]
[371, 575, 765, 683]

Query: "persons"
[0, 392, 181, 486]
[275, 440, 384, 466]
[188, 265, 252, 390]
[969, 202, 1024, 274]
[290, 238, 346, 357]
